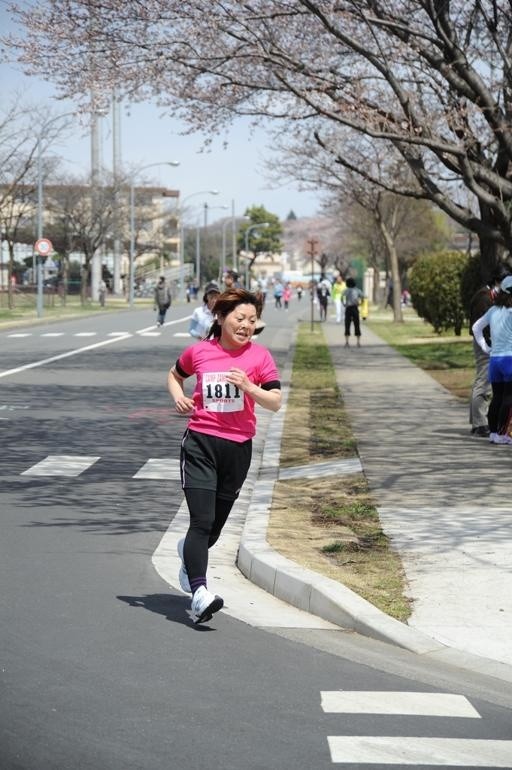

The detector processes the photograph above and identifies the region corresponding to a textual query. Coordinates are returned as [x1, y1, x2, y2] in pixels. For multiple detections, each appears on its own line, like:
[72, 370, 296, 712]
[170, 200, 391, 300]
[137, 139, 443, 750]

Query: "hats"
[500, 275, 511, 294]
[204, 284, 220, 295]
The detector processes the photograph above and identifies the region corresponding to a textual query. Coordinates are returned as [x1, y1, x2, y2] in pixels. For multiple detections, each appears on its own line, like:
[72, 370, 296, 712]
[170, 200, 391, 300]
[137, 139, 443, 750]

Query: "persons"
[186, 270, 266, 342]
[341, 277, 364, 348]
[167, 291, 279, 624]
[471, 276, 512, 446]
[385, 279, 394, 311]
[274, 279, 302, 309]
[101, 280, 105, 306]
[156, 277, 171, 326]
[470, 262, 511, 437]
[309, 276, 348, 322]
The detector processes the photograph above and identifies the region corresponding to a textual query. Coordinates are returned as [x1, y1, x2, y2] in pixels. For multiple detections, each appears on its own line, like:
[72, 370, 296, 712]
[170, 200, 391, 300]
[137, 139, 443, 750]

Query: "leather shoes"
[474, 426, 490, 437]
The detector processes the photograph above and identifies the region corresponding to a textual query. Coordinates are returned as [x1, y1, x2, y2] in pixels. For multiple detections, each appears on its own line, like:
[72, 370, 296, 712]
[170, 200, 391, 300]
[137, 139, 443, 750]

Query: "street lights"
[180, 189, 220, 303]
[37, 107, 109, 318]
[196, 205, 228, 283]
[245, 222, 270, 291]
[129, 160, 180, 309]
[222, 216, 250, 292]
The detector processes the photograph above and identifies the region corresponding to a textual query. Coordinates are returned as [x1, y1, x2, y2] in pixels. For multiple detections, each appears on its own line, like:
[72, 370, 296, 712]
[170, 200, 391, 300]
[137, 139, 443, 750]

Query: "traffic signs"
[35, 239, 53, 256]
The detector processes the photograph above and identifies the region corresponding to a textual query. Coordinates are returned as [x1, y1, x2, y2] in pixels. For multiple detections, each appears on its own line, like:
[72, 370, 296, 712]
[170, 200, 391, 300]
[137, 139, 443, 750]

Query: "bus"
[278, 272, 333, 292]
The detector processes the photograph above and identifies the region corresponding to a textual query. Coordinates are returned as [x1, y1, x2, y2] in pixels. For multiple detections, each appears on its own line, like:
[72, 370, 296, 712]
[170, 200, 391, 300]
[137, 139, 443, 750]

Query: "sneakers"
[490, 432, 495, 442]
[178, 540, 191, 593]
[494, 433, 511, 444]
[190, 586, 223, 624]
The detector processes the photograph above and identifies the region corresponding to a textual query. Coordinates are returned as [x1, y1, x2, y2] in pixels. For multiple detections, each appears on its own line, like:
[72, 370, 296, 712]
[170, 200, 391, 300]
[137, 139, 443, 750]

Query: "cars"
[39, 273, 91, 295]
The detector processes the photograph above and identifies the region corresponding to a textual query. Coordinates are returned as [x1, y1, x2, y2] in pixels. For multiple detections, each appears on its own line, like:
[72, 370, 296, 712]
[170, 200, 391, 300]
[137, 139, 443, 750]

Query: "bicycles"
[126, 279, 180, 298]
